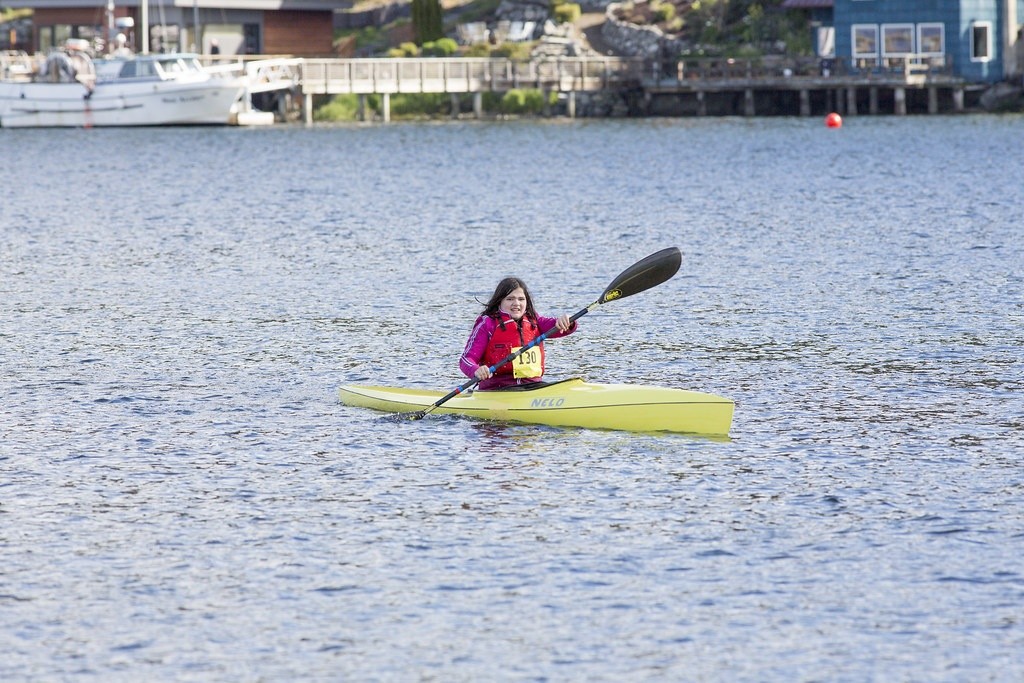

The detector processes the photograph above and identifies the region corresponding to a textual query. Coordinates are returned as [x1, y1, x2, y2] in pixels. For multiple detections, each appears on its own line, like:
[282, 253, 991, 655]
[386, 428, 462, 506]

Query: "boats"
[339, 376, 734, 438]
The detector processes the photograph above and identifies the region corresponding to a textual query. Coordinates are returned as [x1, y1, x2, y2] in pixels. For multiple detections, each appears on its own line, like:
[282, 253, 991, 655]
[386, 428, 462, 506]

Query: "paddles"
[371, 246, 682, 421]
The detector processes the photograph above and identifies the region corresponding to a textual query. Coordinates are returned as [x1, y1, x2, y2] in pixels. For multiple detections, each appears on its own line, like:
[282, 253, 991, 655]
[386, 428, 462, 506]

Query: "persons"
[211, 40, 219, 62]
[460, 277, 577, 390]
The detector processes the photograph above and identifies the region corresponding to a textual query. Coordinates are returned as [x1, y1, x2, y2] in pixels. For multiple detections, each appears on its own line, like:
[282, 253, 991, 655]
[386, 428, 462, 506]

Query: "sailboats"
[0, 0, 306, 126]
